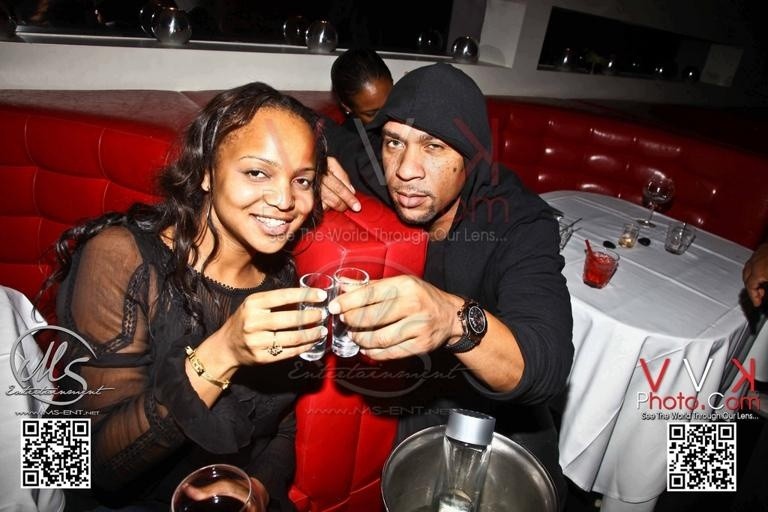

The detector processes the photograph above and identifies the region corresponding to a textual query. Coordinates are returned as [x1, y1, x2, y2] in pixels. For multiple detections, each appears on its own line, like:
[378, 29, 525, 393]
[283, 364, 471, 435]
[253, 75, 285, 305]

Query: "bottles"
[431, 409, 496, 511]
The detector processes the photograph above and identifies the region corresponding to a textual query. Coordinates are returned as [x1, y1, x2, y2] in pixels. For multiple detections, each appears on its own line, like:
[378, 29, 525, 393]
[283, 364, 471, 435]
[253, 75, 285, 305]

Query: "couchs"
[3, 94, 767, 512]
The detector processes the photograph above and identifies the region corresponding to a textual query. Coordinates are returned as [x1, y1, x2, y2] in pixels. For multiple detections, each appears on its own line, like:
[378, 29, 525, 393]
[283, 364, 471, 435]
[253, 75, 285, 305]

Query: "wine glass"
[636, 175, 674, 227]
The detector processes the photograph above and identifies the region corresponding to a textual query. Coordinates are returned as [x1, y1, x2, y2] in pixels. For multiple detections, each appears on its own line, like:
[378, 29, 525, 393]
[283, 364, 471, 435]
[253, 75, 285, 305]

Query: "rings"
[270, 333, 283, 356]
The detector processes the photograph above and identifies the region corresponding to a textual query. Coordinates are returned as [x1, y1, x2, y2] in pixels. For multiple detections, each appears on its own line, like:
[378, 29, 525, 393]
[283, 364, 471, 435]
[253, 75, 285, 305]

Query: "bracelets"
[185, 347, 230, 389]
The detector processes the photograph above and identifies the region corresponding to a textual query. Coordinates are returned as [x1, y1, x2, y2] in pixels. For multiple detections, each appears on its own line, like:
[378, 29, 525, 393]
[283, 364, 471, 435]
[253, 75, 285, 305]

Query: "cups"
[554, 216, 698, 289]
[297, 268, 371, 361]
[171, 464, 258, 512]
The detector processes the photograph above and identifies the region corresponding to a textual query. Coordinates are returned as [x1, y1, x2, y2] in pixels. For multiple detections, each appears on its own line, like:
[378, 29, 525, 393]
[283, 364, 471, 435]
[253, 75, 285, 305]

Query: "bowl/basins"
[379, 423, 560, 512]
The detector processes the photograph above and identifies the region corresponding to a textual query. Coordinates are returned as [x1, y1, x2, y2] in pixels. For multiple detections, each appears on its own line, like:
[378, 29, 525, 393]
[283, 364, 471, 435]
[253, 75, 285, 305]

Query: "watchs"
[444, 294, 488, 355]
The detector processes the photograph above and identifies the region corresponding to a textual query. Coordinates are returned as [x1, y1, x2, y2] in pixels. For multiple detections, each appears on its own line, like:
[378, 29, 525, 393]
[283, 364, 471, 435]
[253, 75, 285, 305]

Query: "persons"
[58, 82, 328, 512]
[321, 63, 574, 494]
[742, 241, 768, 309]
[331, 44, 394, 130]
